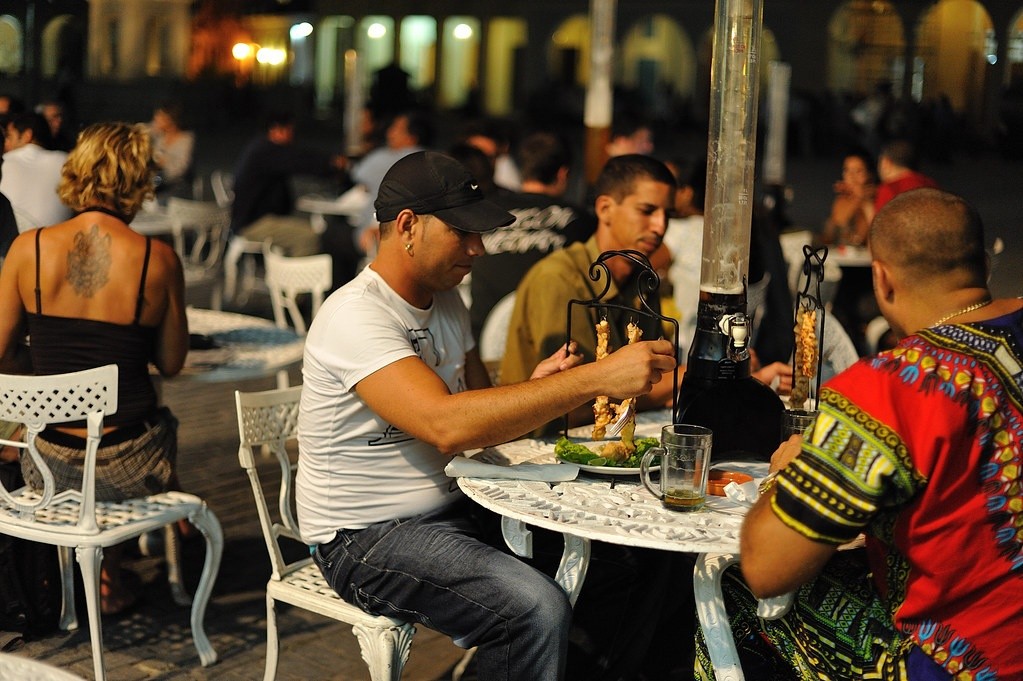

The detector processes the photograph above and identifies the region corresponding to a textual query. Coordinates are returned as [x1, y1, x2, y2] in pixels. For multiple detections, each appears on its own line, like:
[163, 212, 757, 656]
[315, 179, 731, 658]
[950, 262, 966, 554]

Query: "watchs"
[757, 469, 784, 495]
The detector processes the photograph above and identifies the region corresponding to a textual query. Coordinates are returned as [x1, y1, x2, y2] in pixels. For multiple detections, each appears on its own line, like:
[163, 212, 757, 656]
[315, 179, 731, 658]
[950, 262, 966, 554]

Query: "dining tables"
[454, 408, 791, 681]
[142, 304, 309, 612]
[816, 239, 875, 268]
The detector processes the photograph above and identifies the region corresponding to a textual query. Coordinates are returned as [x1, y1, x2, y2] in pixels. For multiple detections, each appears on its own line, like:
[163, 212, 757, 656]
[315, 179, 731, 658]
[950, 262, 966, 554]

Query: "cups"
[640, 424, 713, 513]
[782, 410, 819, 441]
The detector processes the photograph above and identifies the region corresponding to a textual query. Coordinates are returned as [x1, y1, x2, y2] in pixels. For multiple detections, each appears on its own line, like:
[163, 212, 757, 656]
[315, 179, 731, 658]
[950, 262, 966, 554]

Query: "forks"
[610, 336, 663, 436]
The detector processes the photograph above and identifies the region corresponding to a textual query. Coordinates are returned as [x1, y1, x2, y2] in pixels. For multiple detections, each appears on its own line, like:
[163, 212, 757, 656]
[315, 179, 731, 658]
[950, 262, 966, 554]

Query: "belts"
[38, 412, 162, 449]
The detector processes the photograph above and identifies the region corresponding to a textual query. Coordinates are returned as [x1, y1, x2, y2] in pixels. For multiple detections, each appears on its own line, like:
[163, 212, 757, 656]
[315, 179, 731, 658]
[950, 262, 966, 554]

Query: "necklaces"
[934, 298, 991, 326]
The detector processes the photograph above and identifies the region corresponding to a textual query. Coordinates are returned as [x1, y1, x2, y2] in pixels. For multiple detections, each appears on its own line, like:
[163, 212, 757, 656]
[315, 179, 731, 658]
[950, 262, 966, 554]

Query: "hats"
[374, 151, 517, 233]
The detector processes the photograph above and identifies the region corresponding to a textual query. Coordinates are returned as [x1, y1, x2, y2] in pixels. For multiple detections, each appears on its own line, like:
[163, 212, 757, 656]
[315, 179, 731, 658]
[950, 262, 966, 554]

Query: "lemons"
[588, 457, 607, 466]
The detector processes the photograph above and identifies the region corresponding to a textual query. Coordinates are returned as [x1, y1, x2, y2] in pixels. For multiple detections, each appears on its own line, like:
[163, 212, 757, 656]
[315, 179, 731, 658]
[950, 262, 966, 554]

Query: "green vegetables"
[554, 435, 662, 468]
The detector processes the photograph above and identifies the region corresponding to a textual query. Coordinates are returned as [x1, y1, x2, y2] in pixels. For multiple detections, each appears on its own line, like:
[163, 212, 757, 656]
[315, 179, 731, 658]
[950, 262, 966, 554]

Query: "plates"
[556, 459, 662, 475]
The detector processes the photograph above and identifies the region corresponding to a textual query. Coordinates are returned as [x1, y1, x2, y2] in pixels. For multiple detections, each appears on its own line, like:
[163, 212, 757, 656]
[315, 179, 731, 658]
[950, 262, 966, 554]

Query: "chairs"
[235, 384, 415, 681]
[0, 363, 223, 681]
[141, 162, 334, 390]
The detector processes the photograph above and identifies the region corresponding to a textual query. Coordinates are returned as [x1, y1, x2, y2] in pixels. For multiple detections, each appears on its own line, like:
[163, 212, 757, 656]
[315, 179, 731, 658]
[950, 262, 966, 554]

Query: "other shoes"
[103, 599, 143, 618]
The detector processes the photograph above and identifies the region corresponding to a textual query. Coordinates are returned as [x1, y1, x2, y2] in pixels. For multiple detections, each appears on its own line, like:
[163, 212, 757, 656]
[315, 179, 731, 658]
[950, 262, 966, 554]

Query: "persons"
[0, 44, 953, 681]
[694, 186, 1023, 681]
